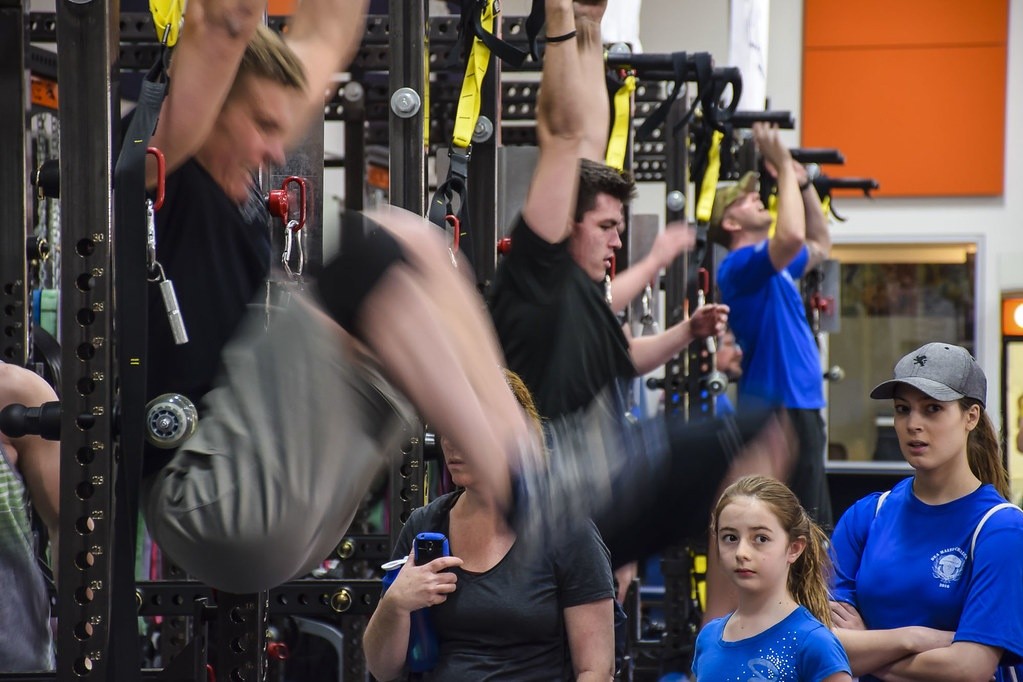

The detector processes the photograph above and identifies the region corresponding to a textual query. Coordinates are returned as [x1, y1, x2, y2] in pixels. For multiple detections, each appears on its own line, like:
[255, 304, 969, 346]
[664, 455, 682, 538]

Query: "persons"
[708, 120, 834, 535]
[0, 358, 62, 675]
[825, 341, 1023, 682]
[488, 0, 799, 639]
[112, 0, 668, 595]
[689, 474, 852, 682]
[360, 365, 616, 682]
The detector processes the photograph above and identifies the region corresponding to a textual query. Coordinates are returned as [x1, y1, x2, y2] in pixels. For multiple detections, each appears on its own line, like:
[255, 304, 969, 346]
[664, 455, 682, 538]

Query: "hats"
[870, 342, 987, 408]
[711, 171, 759, 225]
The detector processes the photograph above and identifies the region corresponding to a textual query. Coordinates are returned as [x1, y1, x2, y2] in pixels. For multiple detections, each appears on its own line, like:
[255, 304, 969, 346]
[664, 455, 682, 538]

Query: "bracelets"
[543, 28, 577, 43]
[800, 179, 812, 192]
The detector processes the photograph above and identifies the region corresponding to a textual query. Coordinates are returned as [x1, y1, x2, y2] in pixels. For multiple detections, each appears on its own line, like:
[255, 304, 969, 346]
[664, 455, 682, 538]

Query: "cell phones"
[413, 533, 450, 573]
